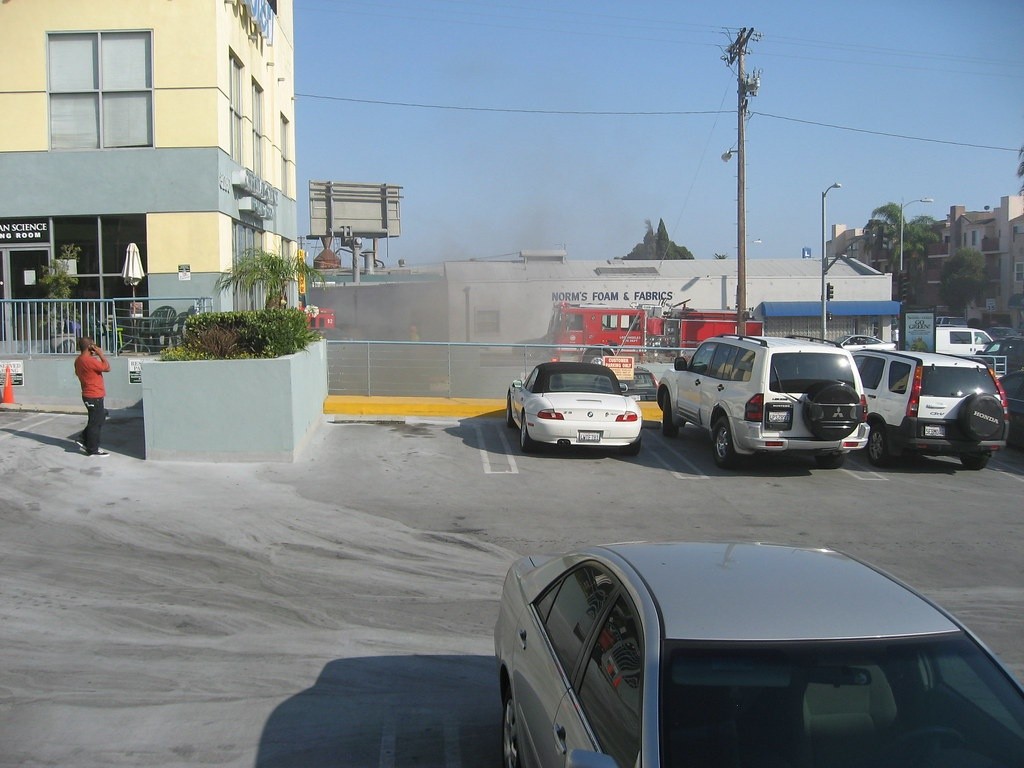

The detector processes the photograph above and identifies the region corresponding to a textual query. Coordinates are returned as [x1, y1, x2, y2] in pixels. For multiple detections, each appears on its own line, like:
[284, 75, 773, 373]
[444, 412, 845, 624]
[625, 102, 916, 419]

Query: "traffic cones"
[1, 365, 15, 404]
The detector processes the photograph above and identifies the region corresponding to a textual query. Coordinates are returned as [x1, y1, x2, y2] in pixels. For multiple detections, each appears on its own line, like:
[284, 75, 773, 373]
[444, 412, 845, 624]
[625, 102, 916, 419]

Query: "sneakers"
[75, 440, 87, 450]
[88, 449, 111, 457]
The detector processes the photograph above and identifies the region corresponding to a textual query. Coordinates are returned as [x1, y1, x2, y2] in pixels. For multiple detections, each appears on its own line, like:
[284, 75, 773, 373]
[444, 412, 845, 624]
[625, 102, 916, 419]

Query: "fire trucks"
[543, 299, 764, 363]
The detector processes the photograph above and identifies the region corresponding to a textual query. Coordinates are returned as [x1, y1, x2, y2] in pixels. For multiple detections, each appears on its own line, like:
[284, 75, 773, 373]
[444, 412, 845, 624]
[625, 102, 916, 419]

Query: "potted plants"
[37, 241, 82, 353]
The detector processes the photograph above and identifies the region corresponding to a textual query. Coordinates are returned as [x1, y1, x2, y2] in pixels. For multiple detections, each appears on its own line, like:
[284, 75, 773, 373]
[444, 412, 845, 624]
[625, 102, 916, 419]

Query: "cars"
[506, 362, 643, 456]
[495, 540, 1024, 768]
[984, 327, 1022, 341]
[832, 334, 897, 352]
[998, 371, 1024, 448]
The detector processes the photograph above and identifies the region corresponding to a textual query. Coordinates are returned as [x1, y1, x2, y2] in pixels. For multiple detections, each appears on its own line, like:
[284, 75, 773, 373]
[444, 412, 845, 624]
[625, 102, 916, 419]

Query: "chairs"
[90, 304, 197, 354]
[801, 650, 899, 768]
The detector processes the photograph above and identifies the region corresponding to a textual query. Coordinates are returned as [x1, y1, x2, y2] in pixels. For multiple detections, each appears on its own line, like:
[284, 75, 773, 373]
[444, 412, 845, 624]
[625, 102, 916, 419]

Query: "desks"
[117, 316, 158, 353]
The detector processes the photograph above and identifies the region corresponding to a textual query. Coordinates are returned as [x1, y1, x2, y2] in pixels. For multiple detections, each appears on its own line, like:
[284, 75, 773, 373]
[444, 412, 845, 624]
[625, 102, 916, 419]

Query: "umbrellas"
[121, 242, 145, 314]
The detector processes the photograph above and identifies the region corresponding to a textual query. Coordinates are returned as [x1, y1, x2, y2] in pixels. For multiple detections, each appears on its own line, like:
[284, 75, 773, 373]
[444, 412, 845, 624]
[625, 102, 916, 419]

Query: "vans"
[936, 316, 993, 356]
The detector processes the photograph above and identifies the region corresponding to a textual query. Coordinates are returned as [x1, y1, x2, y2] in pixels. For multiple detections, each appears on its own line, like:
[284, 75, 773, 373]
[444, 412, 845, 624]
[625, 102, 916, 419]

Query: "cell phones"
[88, 346, 95, 351]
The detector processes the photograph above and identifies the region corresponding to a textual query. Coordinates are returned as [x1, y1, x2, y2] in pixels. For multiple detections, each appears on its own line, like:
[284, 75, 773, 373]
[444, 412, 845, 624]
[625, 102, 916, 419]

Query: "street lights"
[900, 198, 932, 303]
[821, 183, 842, 343]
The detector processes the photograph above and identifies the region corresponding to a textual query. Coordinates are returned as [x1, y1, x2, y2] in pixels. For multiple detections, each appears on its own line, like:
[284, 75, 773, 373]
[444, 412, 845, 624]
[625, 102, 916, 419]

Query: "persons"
[74, 338, 111, 457]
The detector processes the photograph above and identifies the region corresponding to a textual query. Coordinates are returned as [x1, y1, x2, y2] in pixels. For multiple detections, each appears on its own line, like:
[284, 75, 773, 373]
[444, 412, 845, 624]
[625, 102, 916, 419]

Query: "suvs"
[619, 367, 658, 402]
[656, 333, 872, 469]
[851, 349, 1009, 472]
